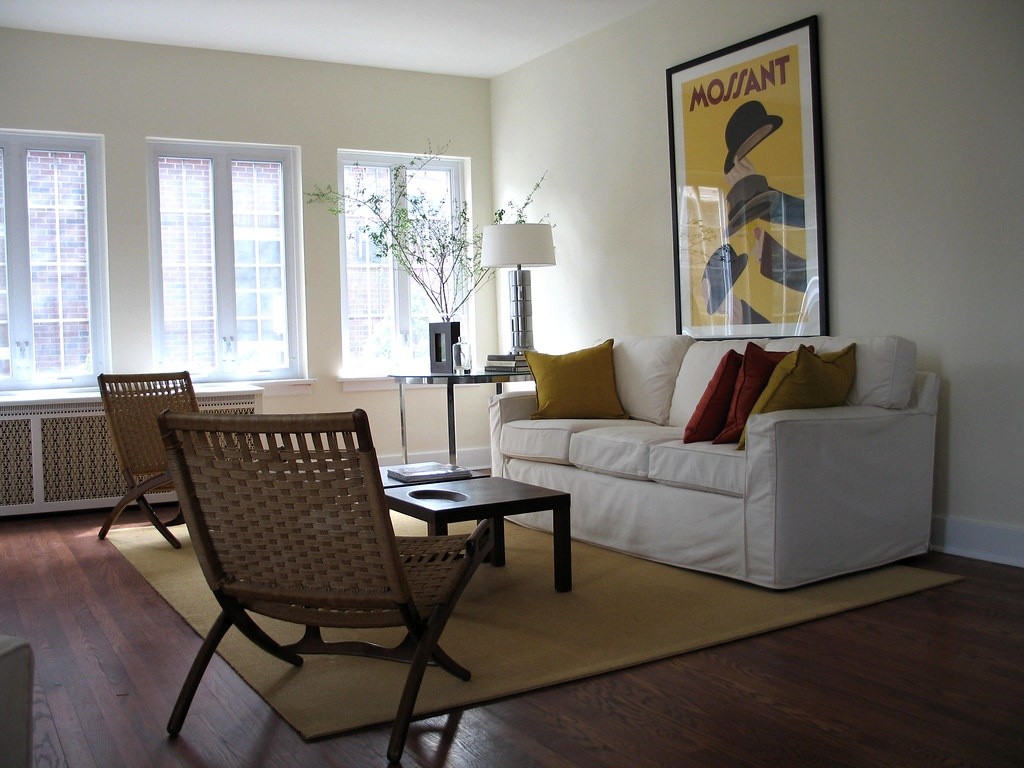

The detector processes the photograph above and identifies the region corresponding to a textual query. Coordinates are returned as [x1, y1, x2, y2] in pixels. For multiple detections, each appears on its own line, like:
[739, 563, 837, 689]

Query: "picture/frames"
[666, 15, 829, 341]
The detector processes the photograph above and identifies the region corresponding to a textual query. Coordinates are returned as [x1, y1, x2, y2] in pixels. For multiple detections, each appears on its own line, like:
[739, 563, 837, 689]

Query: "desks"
[382, 372, 521, 466]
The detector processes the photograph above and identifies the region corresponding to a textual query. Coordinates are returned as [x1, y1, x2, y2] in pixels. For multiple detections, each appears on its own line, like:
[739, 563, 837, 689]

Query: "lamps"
[481, 223, 556, 355]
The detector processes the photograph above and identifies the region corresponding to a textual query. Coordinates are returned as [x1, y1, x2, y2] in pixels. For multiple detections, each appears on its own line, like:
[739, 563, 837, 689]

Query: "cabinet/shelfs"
[0, 385, 265, 516]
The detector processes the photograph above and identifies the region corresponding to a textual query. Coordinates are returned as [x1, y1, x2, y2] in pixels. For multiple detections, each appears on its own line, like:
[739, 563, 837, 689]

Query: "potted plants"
[306, 136, 558, 376]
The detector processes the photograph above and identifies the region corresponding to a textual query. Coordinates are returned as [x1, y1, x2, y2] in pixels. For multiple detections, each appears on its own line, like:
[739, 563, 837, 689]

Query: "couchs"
[489, 334, 941, 590]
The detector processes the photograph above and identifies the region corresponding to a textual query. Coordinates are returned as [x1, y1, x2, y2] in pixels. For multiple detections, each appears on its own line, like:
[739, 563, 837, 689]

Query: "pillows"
[524, 338, 631, 419]
[712, 342, 814, 443]
[737, 342, 857, 451]
[682, 349, 744, 444]
[820, 335, 918, 408]
[765, 336, 830, 353]
[668, 337, 770, 428]
[593, 334, 696, 427]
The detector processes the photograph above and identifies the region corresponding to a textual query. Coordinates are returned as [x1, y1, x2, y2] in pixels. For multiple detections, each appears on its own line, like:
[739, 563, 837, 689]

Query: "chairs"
[97, 372, 202, 549]
[154, 408, 496, 760]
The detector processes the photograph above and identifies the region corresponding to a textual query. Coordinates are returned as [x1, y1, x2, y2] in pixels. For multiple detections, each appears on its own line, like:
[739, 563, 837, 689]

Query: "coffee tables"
[380, 462, 492, 537]
[383, 476, 574, 591]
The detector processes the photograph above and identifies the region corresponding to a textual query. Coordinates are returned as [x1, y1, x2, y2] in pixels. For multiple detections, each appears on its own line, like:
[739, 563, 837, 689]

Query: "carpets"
[105, 491, 966, 740]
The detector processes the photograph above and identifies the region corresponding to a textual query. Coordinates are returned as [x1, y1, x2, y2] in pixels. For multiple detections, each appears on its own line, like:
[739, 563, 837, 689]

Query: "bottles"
[453, 337, 471, 374]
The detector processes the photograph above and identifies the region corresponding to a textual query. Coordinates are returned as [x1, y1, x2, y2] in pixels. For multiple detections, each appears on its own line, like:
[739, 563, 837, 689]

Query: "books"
[387, 463, 472, 484]
[484, 354, 531, 373]
